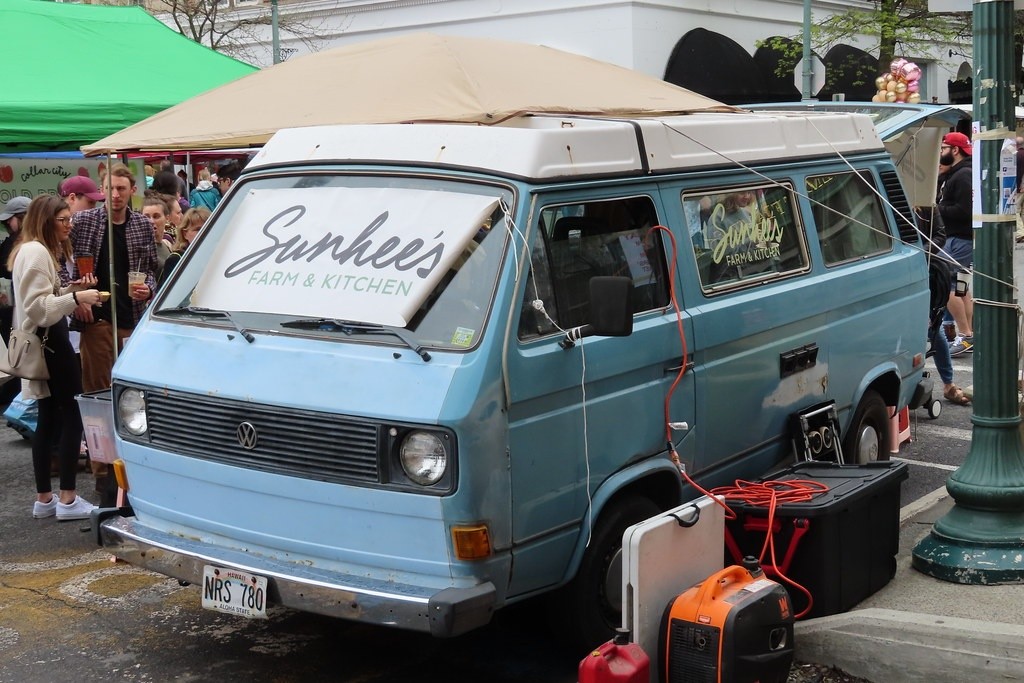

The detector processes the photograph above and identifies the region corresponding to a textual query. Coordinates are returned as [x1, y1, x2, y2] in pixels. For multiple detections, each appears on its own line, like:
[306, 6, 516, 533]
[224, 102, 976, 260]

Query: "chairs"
[551, 217, 617, 328]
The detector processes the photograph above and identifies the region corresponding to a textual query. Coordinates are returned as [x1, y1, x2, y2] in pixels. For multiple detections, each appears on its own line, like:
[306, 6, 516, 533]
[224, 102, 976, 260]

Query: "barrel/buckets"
[577, 627, 651, 683]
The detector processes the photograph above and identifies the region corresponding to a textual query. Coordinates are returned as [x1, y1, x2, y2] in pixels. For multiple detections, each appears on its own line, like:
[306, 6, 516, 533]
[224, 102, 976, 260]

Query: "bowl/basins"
[98, 291, 112, 302]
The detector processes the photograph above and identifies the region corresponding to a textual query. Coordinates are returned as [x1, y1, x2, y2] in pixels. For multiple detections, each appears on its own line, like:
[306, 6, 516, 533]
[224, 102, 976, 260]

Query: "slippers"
[944, 384, 969, 404]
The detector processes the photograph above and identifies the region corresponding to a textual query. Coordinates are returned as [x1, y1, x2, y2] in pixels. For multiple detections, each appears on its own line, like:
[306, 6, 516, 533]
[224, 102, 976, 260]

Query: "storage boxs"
[73, 387, 121, 464]
[716, 458, 908, 621]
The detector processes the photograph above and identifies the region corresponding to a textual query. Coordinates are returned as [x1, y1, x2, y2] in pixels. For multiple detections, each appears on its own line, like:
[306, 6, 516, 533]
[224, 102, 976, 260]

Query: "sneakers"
[948, 333, 974, 356]
[56, 496, 100, 520]
[33, 495, 60, 520]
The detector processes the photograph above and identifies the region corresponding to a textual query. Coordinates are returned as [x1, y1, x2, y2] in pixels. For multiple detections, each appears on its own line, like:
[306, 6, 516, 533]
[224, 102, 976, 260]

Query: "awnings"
[664, 28, 878, 106]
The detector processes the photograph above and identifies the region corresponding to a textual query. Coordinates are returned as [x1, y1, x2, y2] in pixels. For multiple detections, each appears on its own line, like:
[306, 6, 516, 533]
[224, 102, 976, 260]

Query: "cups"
[128, 272, 148, 298]
[76, 253, 94, 279]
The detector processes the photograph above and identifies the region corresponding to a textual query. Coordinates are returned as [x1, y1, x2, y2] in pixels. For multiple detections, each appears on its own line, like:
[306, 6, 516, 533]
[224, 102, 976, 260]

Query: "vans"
[89, 99, 972, 656]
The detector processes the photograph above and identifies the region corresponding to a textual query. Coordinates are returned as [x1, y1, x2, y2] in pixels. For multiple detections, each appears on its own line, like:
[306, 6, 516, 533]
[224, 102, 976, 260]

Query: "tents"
[0, 0, 261, 143]
[83, 34, 742, 361]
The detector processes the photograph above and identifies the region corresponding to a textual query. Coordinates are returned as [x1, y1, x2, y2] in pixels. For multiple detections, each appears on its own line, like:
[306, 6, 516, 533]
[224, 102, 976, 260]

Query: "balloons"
[872, 58, 922, 104]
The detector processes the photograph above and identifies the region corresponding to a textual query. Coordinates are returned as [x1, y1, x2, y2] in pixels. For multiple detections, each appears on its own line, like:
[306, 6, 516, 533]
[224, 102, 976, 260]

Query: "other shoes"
[1016, 236, 1024, 241]
[944, 325, 956, 341]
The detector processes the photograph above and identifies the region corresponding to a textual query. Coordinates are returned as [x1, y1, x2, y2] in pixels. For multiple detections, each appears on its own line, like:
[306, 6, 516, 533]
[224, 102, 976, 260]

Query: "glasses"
[217, 178, 235, 186]
[57, 218, 73, 228]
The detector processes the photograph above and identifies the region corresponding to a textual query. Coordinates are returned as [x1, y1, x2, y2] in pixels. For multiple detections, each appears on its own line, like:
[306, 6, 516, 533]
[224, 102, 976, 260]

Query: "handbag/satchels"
[0, 328, 50, 381]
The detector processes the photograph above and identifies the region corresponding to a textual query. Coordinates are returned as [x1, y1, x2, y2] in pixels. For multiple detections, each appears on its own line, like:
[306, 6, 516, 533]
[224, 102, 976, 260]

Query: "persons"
[4, 194, 99, 520]
[936, 132, 974, 355]
[706, 190, 752, 249]
[1015, 137, 1024, 242]
[56, 161, 159, 393]
[913, 204, 969, 404]
[140, 160, 245, 293]
[0, 196, 32, 349]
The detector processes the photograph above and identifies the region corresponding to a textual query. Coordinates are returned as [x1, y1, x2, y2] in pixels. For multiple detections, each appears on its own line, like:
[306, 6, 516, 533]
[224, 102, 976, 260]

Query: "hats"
[942, 132, 972, 156]
[60, 177, 106, 202]
[0, 196, 33, 221]
